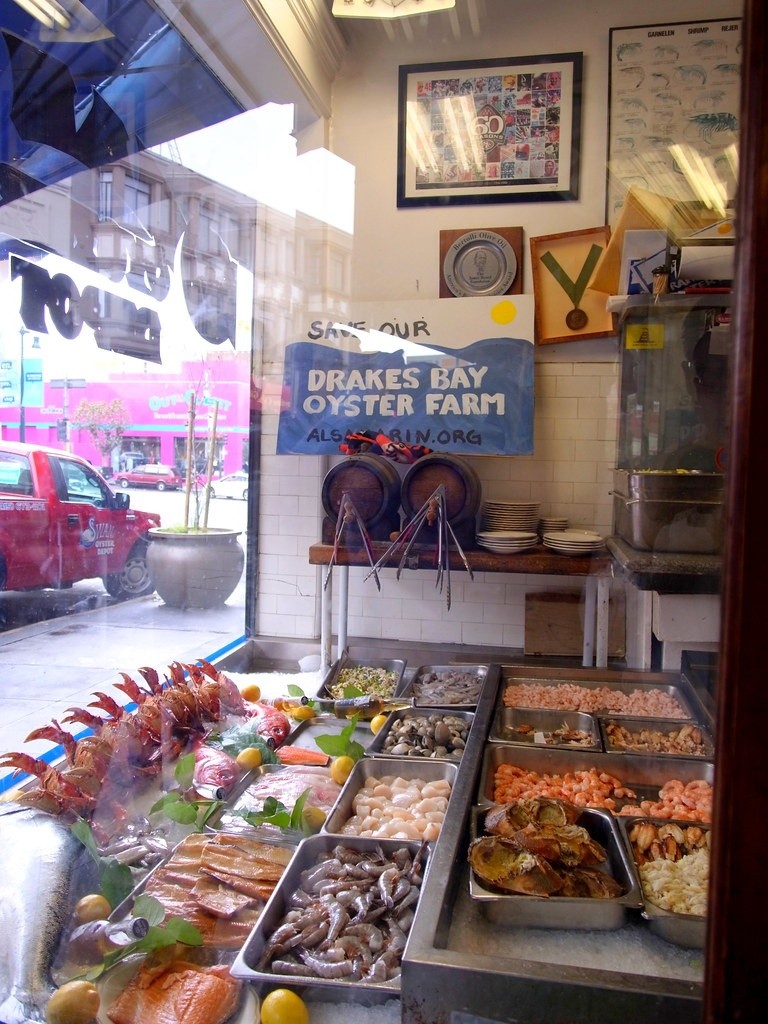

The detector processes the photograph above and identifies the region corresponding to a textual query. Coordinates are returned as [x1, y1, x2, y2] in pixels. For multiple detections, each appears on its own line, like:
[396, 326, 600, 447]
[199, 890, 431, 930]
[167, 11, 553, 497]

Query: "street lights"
[18, 325, 42, 443]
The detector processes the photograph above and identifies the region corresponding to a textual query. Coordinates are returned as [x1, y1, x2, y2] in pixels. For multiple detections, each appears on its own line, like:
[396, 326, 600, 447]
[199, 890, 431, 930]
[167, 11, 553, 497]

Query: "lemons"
[73, 894, 112, 925]
[370, 714, 387, 735]
[241, 685, 260, 702]
[260, 988, 309, 1024]
[331, 755, 356, 785]
[292, 706, 316, 722]
[236, 748, 262, 770]
[304, 807, 327, 829]
[46, 980, 101, 1024]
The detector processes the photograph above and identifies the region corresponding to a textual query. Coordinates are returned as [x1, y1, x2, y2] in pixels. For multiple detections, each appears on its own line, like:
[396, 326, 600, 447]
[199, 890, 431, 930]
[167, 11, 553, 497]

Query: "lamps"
[14, 0, 73, 30]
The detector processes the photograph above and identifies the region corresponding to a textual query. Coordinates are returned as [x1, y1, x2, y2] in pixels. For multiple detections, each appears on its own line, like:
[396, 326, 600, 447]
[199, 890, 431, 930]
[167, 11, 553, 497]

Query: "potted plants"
[145, 525, 244, 609]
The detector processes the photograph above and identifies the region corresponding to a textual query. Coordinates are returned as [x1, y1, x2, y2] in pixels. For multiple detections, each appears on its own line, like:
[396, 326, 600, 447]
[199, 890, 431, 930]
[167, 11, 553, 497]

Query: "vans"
[120, 452, 144, 462]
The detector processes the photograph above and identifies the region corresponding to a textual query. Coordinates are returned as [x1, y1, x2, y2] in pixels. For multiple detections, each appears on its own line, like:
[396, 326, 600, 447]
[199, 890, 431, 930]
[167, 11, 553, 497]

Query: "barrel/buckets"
[399, 451, 482, 531]
[321, 451, 402, 532]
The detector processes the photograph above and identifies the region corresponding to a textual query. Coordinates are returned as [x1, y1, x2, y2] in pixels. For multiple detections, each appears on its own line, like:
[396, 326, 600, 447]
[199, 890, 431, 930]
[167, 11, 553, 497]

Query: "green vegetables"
[313, 710, 365, 761]
[286, 683, 366, 712]
[149, 752, 311, 833]
[75, 820, 206, 980]
[203, 730, 282, 764]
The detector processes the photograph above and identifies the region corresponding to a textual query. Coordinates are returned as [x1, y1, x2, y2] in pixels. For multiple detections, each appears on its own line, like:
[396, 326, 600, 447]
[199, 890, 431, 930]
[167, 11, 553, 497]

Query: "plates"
[539, 517, 607, 555]
[476, 531, 538, 555]
[480, 499, 541, 533]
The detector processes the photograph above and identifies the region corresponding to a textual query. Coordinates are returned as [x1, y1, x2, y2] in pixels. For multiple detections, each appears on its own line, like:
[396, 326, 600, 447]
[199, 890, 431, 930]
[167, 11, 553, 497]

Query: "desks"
[310, 542, 615, 670]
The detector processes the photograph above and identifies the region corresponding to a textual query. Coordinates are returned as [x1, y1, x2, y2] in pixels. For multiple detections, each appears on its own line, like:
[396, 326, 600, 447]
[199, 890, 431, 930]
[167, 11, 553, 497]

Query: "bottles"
[334, 697, 418, 719]
[258, 695, 309, 712]
[159, 776, 227, 801]
[48, 917, 150, 989]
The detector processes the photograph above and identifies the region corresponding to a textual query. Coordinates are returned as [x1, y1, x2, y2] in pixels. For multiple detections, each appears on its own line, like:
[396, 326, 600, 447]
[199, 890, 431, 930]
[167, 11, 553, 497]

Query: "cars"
[207, 471, 249, 501]
[115, 464, 183, 492]
[174, 457, 224, 477]
[0, 441, 163, 601]
[66, 464, 114, 482]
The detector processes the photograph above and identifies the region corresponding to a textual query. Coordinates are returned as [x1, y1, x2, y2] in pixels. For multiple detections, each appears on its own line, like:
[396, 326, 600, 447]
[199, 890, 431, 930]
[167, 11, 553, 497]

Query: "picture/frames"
[396, 50, 585, 207]
[530, 224, 620, 346]
